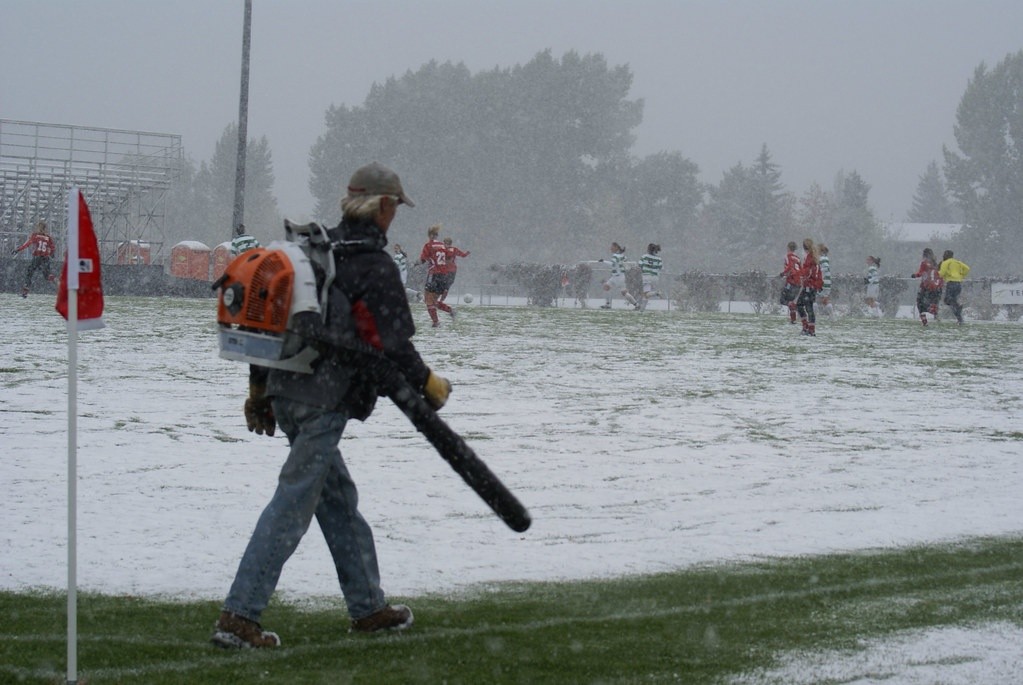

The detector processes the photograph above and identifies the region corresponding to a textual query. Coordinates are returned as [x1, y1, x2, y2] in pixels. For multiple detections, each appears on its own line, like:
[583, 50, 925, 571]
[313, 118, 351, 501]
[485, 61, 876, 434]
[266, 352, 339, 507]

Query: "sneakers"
[351, 603, 414, 633]
[209, 609, 280, 649]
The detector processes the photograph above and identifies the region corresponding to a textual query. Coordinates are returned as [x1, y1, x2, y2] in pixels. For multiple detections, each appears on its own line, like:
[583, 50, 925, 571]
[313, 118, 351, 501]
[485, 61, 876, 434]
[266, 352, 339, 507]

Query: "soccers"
[463, 293, 474, 303]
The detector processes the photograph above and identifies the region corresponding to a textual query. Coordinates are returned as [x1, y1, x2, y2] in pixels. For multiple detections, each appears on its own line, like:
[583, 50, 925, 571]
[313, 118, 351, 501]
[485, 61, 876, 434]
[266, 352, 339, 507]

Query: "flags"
[55, 188, 105, 329]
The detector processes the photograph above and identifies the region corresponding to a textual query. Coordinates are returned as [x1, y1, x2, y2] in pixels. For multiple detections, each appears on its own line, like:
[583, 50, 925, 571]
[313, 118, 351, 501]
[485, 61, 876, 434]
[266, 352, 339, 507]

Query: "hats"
[348, 161, 416, 208]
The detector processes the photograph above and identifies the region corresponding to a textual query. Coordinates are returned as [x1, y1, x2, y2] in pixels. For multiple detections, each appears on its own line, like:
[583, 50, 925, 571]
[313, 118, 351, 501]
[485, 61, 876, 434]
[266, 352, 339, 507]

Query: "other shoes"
[432, 322, 442, 327]
[635, 305, 640, 310]
[657, 289, 665, 299]
[600, 305, 611, 309]
[807, 332, 816, 337]
[800, 330, 808, 336]
[450, 308, 457, 316]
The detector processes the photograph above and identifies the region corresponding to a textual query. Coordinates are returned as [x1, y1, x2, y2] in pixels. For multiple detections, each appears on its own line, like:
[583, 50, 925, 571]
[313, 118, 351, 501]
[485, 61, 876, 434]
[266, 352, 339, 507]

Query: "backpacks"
[211, 213, 397, 375]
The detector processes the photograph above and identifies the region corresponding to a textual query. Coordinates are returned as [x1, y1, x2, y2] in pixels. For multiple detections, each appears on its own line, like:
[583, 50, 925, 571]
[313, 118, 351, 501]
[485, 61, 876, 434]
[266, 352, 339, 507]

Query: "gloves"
[408, 355, 453, 411]
[244, 365, 275, 438]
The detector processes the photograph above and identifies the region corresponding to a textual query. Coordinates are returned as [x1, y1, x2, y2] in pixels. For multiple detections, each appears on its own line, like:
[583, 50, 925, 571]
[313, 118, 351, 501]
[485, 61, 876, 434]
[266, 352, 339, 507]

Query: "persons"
[229, 224, 265, 263]
[795, 237, 825, 338]
[411, 225, 458, 328]
[390, 244, 410, 290]
[859, 246, 970, 327]
[812, 246, 839, 325]
[440, 237, 471, 303]
[210, 163, 453, 654]
[12, 222, 58, 299]
[778, 241, 800, 325]
[637, 243, 665, 312]
[598, 242, 640, 312]
